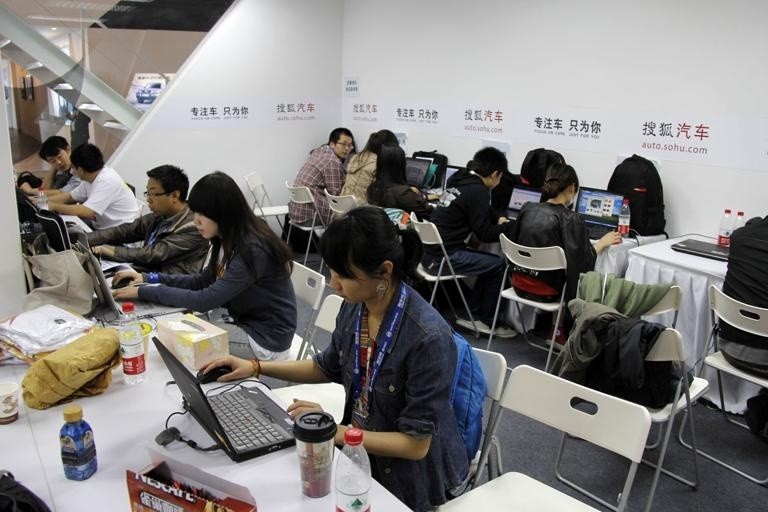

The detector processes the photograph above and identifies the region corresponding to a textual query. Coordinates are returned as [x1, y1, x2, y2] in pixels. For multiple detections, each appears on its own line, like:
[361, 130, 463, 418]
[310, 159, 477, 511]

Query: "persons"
[19, 135, 83, 197]
[506, 160, 623, 352]
[195, 206, 471, 512]
[419, 146, 519, 339]
[716, 215, 768, 440]
[65, 107, 91, 154]
[86, 163, 210, 275]
[30, 143, 140, 249]
[111, 170, 298, 361]
[331, 129, 399, 222]
[287, 128, 354, 228]
[364, 141, 440, 284]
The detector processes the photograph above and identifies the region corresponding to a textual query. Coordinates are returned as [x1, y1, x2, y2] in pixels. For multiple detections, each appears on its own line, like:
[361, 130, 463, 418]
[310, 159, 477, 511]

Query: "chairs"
[573, 270, 684, 454]
[279, 181, 327, 267]
[290, 292, 348, 382]
[280, 258, 328, 370]
[449, 345, 508, 494]
[433, 364, 655, 512]
[552, 298, 712, 512]
[316, 189, 362, 283]
[676, 281, 767, 487]
[240, 171, 290, 233]
[403, 210, 488, 347]
[480, 230, 576, 388]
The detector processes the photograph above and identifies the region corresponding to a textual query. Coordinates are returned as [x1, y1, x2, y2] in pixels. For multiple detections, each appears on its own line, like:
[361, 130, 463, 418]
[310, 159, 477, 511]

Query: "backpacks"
[520, 148, 566, 188]
[608, 154, 666, 238]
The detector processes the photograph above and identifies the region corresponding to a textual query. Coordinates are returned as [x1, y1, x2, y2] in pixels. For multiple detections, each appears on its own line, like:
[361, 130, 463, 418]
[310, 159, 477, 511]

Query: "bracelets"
[250, 358, 261, 378]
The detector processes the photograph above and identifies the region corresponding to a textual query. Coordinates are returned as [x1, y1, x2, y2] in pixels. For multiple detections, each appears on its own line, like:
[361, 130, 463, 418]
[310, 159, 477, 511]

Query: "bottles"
[59, 406, 98, 481]
[335, 429, 373, 512]
[37, 187, 48, 211]
[718, 209, 746, 247]
[119, 302, 148, 386]
[617, 199, 631, 238]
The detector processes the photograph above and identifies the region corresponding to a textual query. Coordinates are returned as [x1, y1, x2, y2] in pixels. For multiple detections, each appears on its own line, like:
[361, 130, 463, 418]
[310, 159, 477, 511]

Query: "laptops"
[405, 157, 432, 191]
[442, 164, 464, 194]
[573, 186, 625, 242]
[76, 240, 191, 322]
[506, 185, 543, 221]
[74, 223, 125, 272]
[671, 239, 729, 261]
[152, 335, 298, 463]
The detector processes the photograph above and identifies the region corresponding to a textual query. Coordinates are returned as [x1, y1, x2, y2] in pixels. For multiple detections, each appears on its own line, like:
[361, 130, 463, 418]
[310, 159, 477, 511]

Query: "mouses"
[197, 364, 232, 384]
[114, 278, 134, 288]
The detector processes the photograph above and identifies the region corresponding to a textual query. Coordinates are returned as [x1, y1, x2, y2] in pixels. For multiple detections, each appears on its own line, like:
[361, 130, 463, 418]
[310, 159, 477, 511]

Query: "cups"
[1, 383, 19, 424]
[122, 320, 152, 363]
[294, 411, 337, 499]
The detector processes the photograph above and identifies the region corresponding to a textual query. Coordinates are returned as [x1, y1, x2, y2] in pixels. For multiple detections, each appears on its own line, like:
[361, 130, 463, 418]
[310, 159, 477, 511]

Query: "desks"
[0, 315, 414, 512]
[427, 171, 669, 281]
[622, 228, 767, 418]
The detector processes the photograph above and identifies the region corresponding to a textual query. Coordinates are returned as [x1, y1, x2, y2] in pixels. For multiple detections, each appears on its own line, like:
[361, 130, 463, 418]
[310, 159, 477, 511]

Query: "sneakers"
[490, 325, 518, 339]
[546, 324, 568, 352]
[743, 408, 767, 438]
[455, 315, 497, 336]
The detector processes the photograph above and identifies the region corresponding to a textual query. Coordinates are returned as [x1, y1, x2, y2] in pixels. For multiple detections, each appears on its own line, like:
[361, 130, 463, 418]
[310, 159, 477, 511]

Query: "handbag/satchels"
[449, 334, 486, 463]
[409, 150, 448, 185]
[16, 169, 43, 187]
[22, 248, 104, 320]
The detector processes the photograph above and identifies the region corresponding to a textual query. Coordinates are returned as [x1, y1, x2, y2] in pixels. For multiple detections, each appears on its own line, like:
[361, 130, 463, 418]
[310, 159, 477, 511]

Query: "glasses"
[334, 140, 355, 150]
[143, 190, 171, 201]
